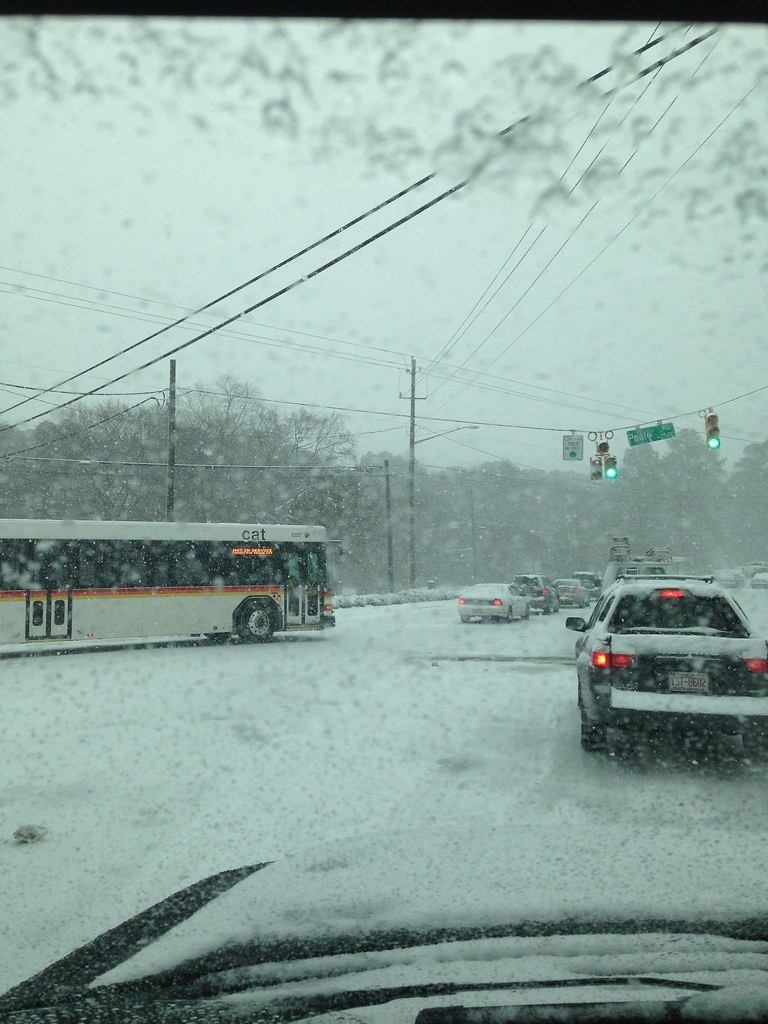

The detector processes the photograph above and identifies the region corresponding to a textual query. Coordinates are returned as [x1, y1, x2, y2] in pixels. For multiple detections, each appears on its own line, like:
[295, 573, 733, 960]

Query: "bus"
[0, 518, 335, 654]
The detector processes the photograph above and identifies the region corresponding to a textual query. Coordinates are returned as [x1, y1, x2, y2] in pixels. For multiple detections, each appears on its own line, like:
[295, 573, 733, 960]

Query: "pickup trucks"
[574, 575, 768, 753]
[741, 562, 768, 576]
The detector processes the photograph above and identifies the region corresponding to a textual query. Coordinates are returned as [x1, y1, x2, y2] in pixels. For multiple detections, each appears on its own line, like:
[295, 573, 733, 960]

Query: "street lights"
[408, 425, 479, 589]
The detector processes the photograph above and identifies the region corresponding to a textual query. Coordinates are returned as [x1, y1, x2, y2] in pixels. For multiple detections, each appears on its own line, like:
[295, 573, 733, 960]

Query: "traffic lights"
[588, 455, 604, 480]
[595, 442, 610, 454]
[603, 454, 618, 480]
[705, 409, 722, 452]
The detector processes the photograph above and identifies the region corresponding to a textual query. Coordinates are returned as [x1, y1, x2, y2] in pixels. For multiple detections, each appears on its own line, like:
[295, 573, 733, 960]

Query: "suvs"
[511, 572, 560, 616]
[571, 572, 602, 600]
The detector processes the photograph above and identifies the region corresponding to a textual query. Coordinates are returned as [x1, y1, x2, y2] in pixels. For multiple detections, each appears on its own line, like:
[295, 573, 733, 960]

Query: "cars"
[551, 579, 590, 608]
[457, 584, 530, 622]
[711, 569, 737, 589]
[751, 573, 768, 588]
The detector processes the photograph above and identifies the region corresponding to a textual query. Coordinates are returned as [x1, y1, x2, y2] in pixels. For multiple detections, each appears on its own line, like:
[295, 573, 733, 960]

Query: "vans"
[602, 538, 675, 594]
